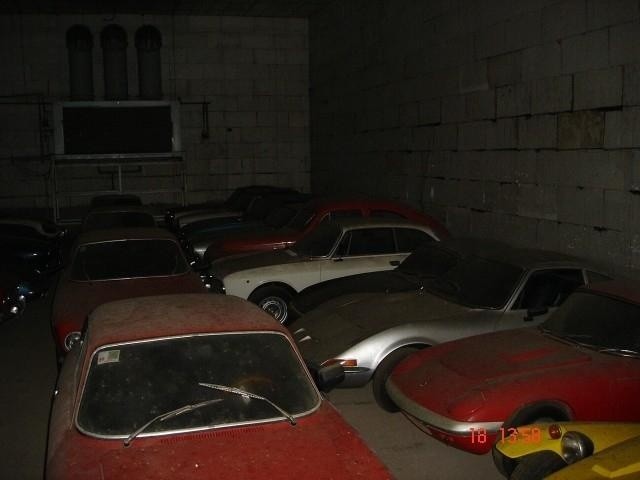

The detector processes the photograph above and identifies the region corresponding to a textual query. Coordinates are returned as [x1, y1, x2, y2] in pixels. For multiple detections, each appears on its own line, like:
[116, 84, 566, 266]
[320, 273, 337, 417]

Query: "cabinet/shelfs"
[49, 152, 185, 226]
